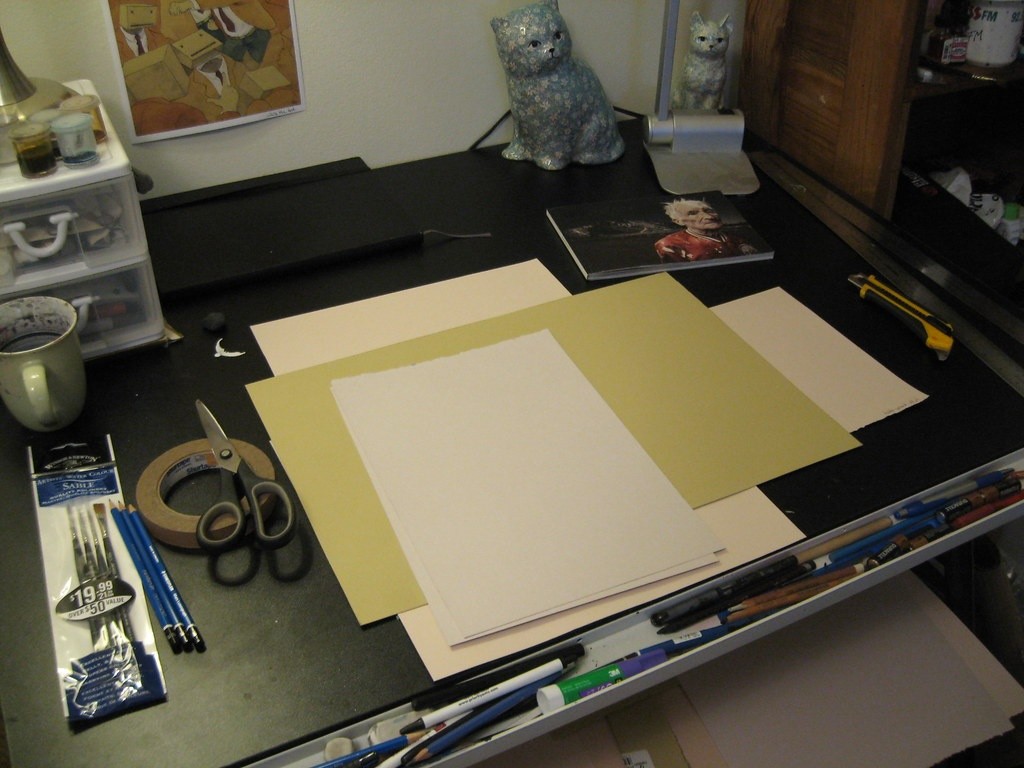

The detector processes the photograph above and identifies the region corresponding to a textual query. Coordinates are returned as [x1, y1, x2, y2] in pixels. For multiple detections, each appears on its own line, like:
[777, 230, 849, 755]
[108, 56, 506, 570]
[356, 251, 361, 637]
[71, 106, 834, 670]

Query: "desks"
[1, 120, 1023, 768]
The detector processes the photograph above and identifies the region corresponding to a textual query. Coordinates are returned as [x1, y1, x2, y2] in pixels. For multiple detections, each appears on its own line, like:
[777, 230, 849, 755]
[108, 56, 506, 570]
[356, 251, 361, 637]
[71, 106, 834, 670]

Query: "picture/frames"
[102, 0, 306, 146]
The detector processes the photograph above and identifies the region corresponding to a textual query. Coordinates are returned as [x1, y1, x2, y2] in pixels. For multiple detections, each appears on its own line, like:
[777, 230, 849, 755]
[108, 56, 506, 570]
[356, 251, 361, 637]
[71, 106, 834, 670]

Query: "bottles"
[965, 0, 1024, 72]
[10, 94, 108, 181]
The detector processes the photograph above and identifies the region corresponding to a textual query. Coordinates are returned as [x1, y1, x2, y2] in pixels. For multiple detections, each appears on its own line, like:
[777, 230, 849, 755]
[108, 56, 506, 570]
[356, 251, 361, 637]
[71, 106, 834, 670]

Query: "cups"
[0, 296, 87, 432]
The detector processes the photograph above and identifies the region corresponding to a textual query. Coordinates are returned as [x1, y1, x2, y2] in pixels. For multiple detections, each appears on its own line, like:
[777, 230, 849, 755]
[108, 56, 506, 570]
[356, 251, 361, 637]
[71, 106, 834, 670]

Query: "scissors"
[192, 398, 299, 554]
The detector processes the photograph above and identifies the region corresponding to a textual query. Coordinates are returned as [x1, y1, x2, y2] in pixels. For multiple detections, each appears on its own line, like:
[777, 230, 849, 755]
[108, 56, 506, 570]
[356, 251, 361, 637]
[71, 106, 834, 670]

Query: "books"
[547, 187, 775, 281]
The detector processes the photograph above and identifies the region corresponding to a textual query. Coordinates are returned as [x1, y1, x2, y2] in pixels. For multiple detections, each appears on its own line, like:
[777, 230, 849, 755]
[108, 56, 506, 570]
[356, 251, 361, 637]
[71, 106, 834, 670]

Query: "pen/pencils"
[306, 467, 1023, 768]
[917, 59, 997, 84]
[106, 499, 208, 654]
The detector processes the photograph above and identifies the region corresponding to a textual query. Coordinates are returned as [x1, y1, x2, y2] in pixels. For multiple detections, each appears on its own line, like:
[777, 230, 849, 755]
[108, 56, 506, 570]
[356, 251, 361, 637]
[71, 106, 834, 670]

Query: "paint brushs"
[63, 500, 134, 651]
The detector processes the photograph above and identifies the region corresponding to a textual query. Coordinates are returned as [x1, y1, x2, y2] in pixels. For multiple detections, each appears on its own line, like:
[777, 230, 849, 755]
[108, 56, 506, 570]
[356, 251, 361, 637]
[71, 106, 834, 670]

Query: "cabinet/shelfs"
[739, 0, 1024, 222]
[1, 78, 164, 361]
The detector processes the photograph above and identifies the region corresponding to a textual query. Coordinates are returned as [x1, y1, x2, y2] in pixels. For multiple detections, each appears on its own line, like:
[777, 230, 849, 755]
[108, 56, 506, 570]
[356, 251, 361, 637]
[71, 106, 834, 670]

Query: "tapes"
[136, 438, 278, 551]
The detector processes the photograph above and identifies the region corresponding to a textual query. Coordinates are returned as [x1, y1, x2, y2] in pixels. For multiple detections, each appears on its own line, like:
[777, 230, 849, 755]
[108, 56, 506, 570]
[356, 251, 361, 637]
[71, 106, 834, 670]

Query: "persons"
[656, 197, 756, 262]
